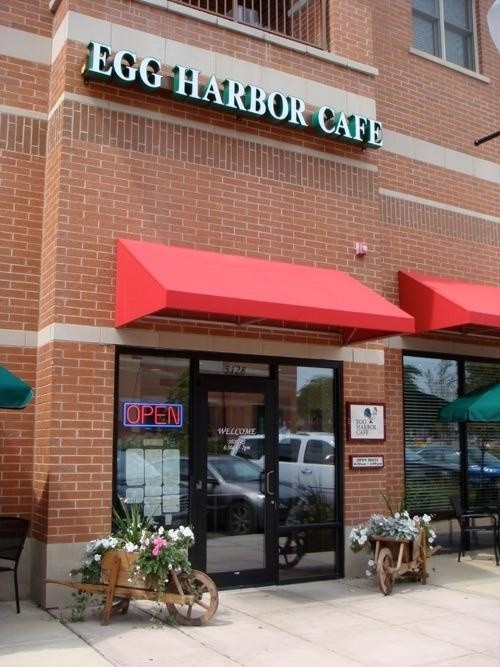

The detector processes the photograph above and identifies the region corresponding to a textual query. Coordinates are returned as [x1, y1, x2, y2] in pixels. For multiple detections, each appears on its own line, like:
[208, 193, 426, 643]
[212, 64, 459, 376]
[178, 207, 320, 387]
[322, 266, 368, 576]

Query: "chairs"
[448, 494, 500, 566]
[0, 516, 31, 613]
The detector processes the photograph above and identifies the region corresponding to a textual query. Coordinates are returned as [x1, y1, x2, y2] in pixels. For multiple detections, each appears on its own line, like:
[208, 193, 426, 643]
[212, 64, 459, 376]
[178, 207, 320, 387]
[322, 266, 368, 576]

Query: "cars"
[117, 431, 500, 534]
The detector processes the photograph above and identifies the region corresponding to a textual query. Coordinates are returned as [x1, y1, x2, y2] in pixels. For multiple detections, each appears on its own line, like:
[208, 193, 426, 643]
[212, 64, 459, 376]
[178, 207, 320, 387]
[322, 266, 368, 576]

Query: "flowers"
[349, 488, 436, 576]
[61, 496, 195, 627]
[285, 478, 335, 525]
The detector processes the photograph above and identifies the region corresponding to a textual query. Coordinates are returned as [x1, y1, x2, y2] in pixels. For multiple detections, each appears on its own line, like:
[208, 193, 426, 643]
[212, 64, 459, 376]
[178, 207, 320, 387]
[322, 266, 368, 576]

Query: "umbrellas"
[435, 379, 500, 424]
[0, 363, 34, 410]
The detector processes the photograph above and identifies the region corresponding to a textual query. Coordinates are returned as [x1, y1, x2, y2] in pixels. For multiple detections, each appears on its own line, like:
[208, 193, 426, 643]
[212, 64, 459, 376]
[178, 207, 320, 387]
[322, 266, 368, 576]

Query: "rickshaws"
[367, 530, 442, 596]
[41, 548, 219, 626]
